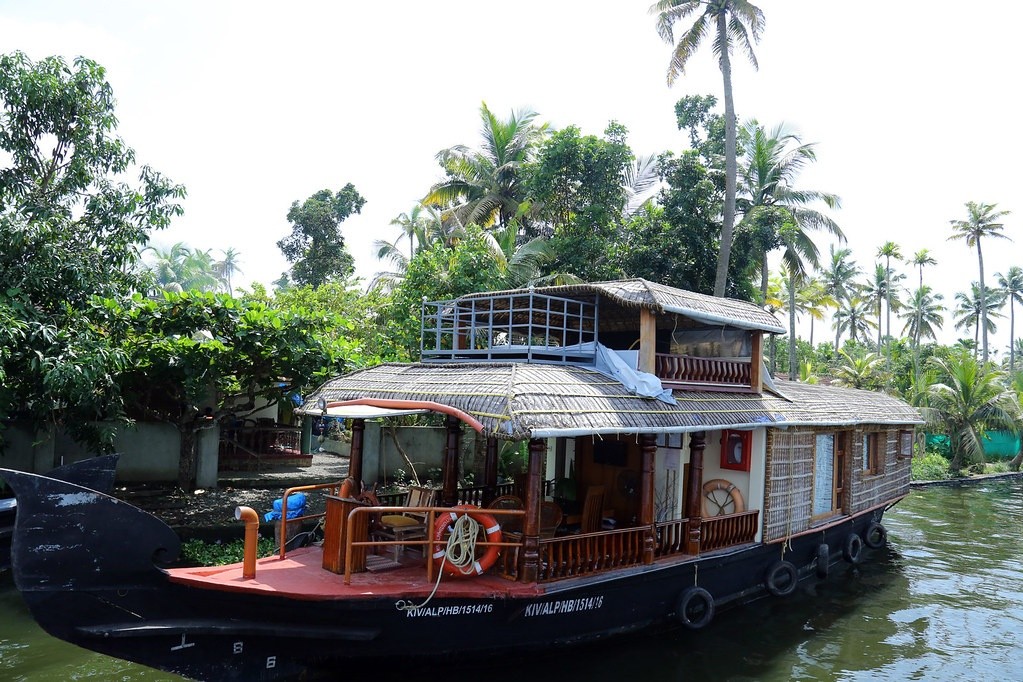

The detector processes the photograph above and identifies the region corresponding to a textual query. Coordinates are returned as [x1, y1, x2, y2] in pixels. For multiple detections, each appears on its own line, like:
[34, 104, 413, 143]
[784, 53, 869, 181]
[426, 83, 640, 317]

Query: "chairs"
[256, 417, 277, 441]
[229, 421, 243, 448]
[540, 502, 563, 554]
[371, 485, 434, 563]
[566, 485, 608, 543]
[487, 495, 525, 519]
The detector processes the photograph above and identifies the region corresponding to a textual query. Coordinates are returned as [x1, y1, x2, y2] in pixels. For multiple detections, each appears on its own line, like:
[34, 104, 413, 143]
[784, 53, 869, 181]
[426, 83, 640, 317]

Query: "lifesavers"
[864, 524, 886, 548]
[817, 544, 829, 575]
[700, 479, 744, 518]
[681, 587, 715, 629]
[842, 534, 861, 563]
[433, 505, 502, 578]
[767, 560, 798, 597]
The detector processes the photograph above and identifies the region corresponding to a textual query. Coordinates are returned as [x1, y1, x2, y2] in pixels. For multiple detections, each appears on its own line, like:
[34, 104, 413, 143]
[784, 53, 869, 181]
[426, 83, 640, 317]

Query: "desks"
[562, 509, 615, 525]
[497, 531, 522, 581]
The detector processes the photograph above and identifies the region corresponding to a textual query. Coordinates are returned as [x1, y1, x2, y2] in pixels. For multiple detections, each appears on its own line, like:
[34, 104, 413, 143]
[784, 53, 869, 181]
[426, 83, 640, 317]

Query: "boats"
[1, 278, 929, 682]
